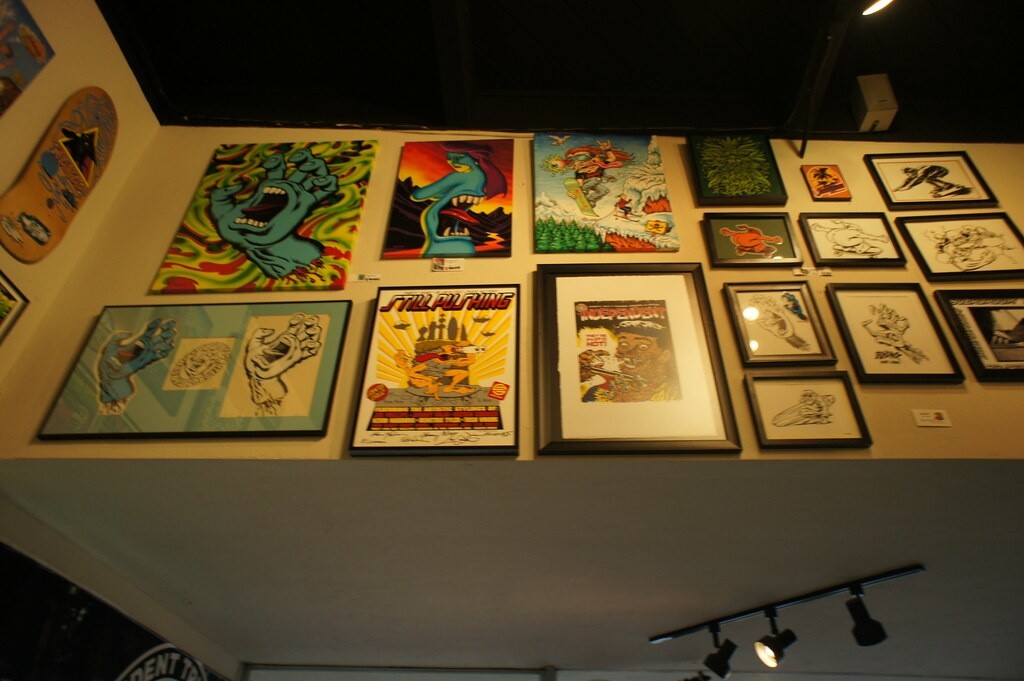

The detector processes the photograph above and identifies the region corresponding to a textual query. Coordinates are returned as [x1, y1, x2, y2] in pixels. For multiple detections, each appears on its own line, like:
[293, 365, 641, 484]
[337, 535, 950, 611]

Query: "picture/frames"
[800, 212, 907, 267]
[745, 367, 871, 450]
[535, 262, 742, 452]
[934, 289, 1024, 382]
[703, 212, 802, 268]
[0, 268, 30, 348]
[35, 300, 348, 441]
[826, 281, 966, 383]
[349, 283, 522, 456]
[863, 149, 999, 211]
[895, 212, 1024, 282]
[724, 280, 839, 366]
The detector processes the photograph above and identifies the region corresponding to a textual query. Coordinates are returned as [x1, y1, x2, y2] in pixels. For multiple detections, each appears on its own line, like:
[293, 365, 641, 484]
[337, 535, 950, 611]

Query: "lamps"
[844, 588, 887, 647]
[703, 623, 738, 679]
[754, 609, 797, 668]
